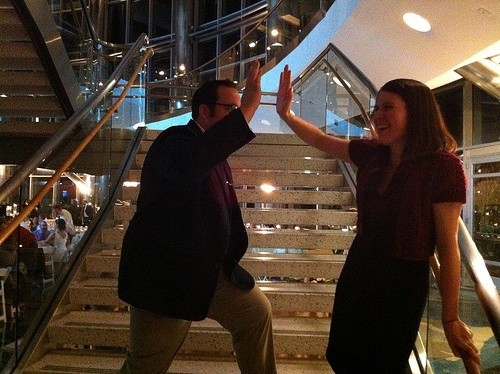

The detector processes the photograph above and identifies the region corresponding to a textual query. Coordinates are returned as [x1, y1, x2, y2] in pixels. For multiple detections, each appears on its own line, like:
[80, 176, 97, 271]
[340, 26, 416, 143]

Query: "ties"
[216, 163, 234, 217]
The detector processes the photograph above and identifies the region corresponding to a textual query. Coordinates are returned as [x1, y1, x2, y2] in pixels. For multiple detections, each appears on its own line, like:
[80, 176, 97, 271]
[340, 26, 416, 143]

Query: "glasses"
[215, 102, 239, 111]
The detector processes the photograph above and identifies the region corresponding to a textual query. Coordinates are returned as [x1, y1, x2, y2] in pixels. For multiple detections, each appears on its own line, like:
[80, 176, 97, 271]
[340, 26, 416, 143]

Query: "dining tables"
[0, 202, 89, 362]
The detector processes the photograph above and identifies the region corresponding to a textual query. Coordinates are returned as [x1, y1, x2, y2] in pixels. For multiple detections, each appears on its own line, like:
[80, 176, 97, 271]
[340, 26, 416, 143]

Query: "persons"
[276, 64, 483, 373]
[2, 202, 97, 310]
[116, 57, 278, 374]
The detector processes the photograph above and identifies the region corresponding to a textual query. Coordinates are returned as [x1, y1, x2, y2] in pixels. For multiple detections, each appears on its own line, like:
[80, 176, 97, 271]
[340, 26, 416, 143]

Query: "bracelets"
[442, 318, 459, 324]
